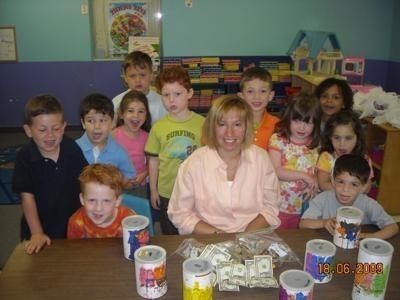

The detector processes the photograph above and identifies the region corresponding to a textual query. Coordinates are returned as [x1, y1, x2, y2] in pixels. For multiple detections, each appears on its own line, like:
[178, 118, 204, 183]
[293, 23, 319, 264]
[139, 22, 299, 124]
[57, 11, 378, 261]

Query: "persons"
[298, 154, 399, 240]
[237, 66, 281, 151]
[266, 91, 322, 229]
[166, 93, 281, 236]
[313, 77, 354, 150]
[315, 110, 375, 195]
[11, 94, 89, 255]
[74, 93, 138, 185]
[111, 50, 169, 236]
[144, 64, 207, 234]
[112, 91, 150, 199]
[66, 163, 137, 240]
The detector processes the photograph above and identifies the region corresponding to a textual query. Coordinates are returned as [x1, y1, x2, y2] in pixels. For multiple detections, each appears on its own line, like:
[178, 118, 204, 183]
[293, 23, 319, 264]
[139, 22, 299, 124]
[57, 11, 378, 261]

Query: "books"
[161, 57, 290, 108]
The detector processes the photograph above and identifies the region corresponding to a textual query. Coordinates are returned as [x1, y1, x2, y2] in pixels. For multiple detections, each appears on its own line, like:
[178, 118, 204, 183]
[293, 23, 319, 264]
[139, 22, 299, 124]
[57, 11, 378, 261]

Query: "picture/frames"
[0, 26, 18, 62]
[91, 0, 162, 58]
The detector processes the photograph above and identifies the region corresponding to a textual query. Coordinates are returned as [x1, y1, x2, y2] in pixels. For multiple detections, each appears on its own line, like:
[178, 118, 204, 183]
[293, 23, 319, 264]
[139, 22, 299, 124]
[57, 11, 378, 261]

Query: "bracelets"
[213, 230, 220, 233]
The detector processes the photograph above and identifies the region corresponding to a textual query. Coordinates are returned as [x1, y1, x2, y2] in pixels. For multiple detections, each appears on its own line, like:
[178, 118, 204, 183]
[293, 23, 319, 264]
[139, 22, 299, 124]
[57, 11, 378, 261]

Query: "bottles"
[121, 206, 394, 300]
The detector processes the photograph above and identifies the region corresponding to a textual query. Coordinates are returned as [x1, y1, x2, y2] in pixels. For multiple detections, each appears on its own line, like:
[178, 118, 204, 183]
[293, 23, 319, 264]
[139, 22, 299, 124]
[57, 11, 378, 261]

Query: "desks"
[0, 220, 400, 300]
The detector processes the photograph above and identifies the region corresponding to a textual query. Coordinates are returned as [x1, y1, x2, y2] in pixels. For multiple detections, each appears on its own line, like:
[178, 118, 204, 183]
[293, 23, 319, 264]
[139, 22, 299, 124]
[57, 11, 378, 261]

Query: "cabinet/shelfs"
[291, 71, 400, 217]
